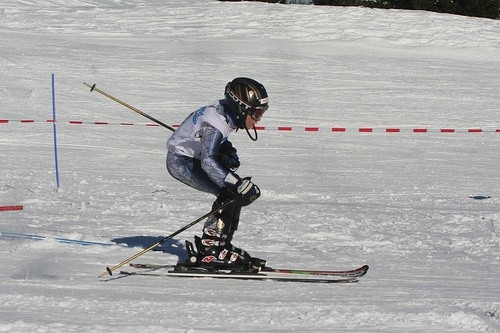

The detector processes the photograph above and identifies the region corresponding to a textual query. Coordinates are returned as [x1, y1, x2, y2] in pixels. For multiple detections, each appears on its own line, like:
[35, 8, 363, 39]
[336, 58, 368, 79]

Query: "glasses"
[246, 104, 266, 120]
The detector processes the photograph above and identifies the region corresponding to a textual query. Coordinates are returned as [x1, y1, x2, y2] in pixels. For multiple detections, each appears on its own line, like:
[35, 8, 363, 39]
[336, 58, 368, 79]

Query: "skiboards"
[117, 260, 370, 283]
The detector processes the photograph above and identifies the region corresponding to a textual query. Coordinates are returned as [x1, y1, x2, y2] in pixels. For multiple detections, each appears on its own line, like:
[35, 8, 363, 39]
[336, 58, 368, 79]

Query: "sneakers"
[199, 234, 250, 271]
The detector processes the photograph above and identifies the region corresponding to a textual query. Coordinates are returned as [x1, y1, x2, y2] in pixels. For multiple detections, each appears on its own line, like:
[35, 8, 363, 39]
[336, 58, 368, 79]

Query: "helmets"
[226, 78, 268, 130]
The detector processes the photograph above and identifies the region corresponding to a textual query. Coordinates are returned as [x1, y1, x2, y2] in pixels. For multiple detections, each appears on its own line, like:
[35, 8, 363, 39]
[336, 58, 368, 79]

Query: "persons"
[164, 76, 269, 271]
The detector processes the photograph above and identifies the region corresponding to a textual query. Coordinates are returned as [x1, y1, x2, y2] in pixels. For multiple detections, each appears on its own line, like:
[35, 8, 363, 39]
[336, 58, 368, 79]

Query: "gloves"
[218, 140, 240, 170]
[232, 176, 260, 206]
